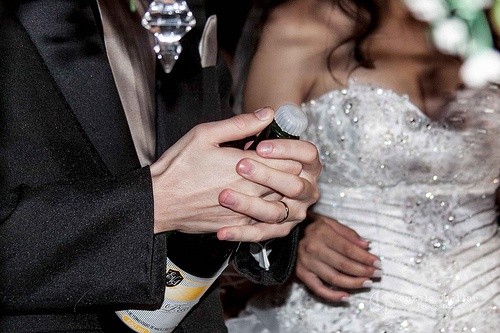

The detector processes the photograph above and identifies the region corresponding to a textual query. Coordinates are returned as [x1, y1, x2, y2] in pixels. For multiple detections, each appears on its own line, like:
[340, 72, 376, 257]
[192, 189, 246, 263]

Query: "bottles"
[100, 105, 306, 333]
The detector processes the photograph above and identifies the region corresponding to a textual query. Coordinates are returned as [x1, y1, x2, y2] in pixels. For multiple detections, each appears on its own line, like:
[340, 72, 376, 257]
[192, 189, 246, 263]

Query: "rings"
[277, 198, 290, 224]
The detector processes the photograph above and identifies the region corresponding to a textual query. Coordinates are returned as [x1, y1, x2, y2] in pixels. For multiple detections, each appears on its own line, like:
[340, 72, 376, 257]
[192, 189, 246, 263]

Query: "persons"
[1, 1, 322, 333]
[242, 2, 500, 333]
